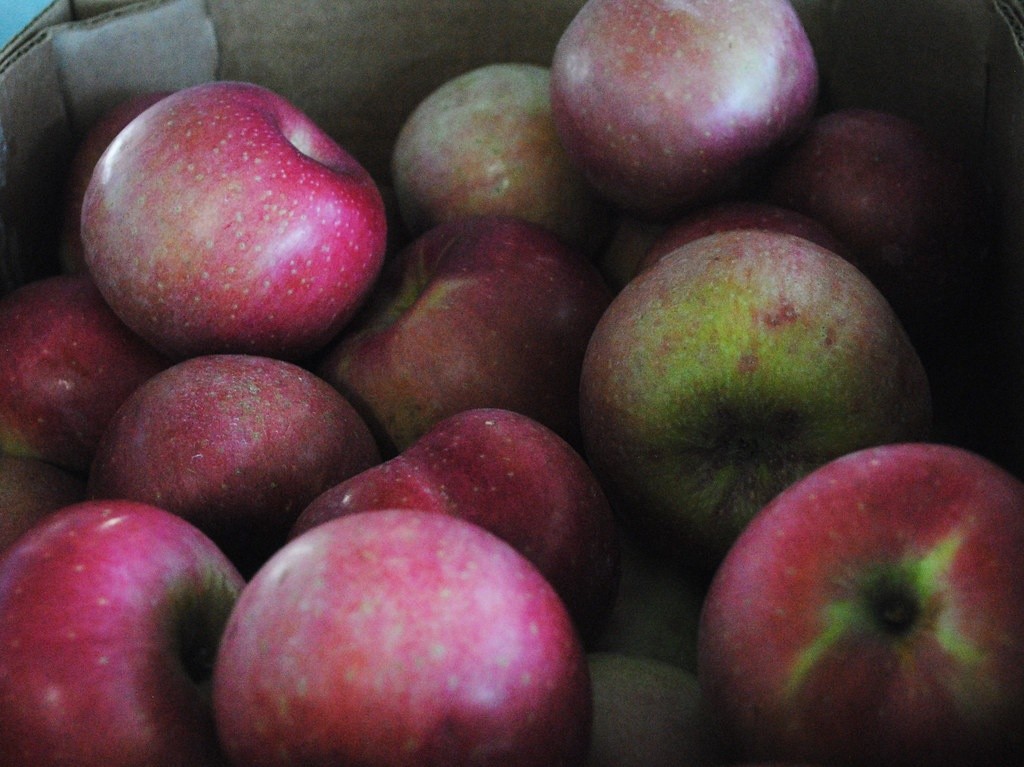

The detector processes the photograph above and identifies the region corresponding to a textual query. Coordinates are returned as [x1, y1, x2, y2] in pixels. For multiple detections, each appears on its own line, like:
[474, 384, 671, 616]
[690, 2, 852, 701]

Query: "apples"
[0, 0, 1023, 765]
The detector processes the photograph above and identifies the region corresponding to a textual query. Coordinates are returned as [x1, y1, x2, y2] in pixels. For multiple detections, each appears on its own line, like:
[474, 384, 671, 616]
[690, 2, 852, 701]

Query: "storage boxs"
[0, 0, 1024, 288]
